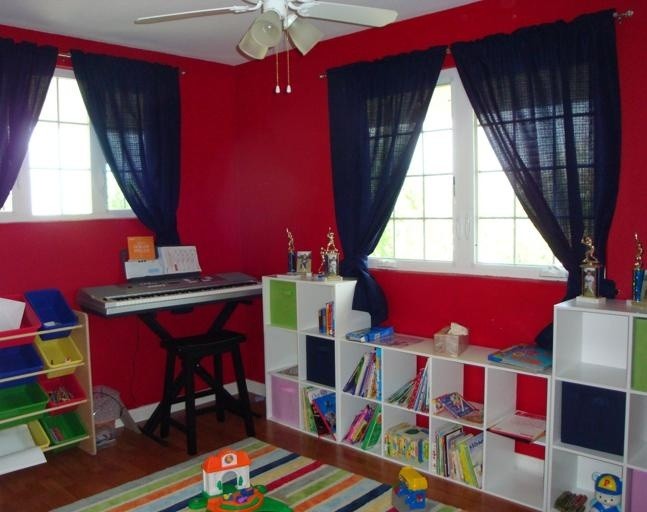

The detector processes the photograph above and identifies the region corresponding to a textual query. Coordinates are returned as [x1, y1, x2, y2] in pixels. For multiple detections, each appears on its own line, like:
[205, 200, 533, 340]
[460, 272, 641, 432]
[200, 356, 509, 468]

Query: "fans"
[132, 0, 399, 45]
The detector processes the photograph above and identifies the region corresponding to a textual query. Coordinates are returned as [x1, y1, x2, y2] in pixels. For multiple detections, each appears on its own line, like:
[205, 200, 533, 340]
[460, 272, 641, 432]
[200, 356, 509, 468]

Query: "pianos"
[74, 272, 261, 319]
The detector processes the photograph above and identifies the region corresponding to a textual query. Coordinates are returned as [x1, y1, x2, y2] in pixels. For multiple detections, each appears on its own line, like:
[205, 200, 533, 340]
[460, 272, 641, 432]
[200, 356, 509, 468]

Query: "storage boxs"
[0, 382, 49, 429]
[0, 344, 42, 389]
[33, 335, 83, 379]
[270, 279, 297, 330]
[40, 409, 88, 444]
[0, 420, 50, 457]
[35, 374, 88, 416]
[25, 288, 77, 341]
[631, 319, 647, 393]
[0, 295, 40, 348]
[563, 382, 624, 456]
[306, 335, 335, 390]
[271, 376, 299, 430]
[628, 469, 647, 512]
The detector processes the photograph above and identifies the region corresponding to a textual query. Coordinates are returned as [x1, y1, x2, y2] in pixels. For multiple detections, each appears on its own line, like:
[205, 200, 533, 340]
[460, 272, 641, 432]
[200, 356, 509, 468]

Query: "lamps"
[238, 10, 325, 60]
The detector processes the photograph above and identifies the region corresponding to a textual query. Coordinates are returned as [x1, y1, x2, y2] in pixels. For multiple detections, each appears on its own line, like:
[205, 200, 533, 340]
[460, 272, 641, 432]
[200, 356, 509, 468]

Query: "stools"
[159, 329, 256, 455]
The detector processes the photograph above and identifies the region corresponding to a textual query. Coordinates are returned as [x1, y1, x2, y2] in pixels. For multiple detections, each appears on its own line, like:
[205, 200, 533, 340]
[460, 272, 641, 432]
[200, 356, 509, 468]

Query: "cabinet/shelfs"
[1, 307, 97, 472]
[262, 272, 647, 512]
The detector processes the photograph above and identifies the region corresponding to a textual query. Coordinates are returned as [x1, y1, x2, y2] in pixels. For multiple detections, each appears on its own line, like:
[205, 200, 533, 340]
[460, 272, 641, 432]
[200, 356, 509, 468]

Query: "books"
[390, 364, 431, 412]
[487, 414, 547, 442]
[346, 348, 381, 400]
[317, 300, 335, 337]
[345, 404, 383, 449]
[434, 392, 484, 425]
[436, 426, 484, 487]
[302, 386, 336, 439]
[384, 422, 430, 466]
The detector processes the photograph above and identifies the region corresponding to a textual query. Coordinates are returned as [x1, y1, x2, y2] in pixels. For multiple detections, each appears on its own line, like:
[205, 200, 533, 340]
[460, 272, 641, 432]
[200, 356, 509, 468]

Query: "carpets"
[45, 435, 469, 512]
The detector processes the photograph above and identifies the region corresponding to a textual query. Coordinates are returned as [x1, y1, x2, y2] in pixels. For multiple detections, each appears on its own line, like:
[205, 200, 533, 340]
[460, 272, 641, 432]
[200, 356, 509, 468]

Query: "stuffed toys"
[591, 472, 622, 511]
